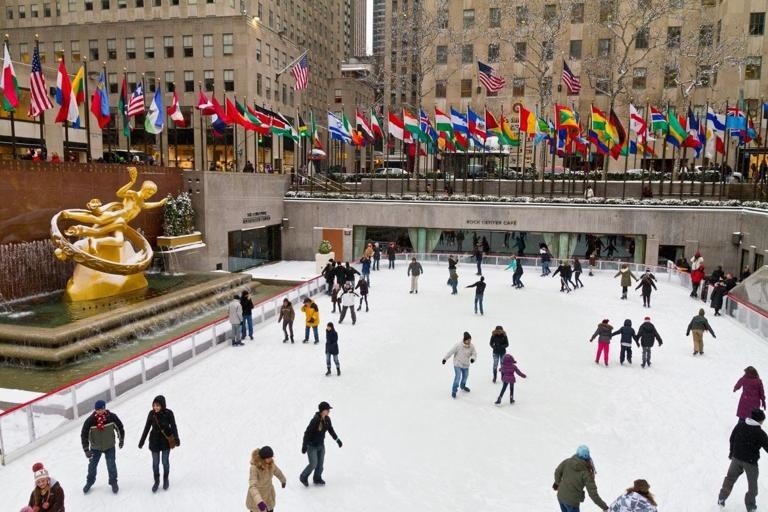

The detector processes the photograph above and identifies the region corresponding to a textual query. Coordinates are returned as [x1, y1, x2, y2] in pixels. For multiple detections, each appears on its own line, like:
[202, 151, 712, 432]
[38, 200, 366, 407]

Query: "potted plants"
[318, 240, 332, 254]
[157, 191, 202, 248]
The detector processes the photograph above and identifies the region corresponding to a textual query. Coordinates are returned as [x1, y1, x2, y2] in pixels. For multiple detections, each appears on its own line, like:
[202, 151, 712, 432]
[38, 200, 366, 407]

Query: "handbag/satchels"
[164, 432, 177, 449]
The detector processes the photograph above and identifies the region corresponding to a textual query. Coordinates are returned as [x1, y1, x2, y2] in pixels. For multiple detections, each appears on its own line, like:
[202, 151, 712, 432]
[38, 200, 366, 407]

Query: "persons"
[209, 158, 255, 174]
[584, 185, 594, 199]
[688, 162, 768, 181]
[642, 185, 653, 200]
[427, 181, 453, 196]
[62, 167, 169, 255]
[433, 230, 635, 261]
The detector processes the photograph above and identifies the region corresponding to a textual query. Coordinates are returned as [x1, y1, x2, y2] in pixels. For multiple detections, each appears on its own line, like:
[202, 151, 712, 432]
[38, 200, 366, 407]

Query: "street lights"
[301, 132, 306, 170]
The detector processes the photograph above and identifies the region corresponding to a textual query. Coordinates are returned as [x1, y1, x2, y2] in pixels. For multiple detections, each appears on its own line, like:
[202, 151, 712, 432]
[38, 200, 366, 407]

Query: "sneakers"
[595, 351, 705, 368]
[231, 307, 369, 378]
[451, 377, 517, 405]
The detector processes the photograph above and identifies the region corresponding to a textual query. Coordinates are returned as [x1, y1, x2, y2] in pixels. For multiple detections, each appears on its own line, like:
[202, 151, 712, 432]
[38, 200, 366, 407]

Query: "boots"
[152, 474, 159, 491]
[82, 481, 94, 493]
[746, 501, 757, 512]
[111, 481, 119, 493]
[313, 476, 325, 484]
[163, 477, 169, 490]
[718, 498, 726, 506]
[299, 474, 309, 486]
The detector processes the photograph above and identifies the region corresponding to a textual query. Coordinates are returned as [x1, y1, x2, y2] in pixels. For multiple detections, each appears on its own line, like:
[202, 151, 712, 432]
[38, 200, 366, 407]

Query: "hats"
[94, 400, 106, 411]
[644, 317, 651, 320]
[463, 332, 472, 342]
[752, 408, 765, 423]
[699, 308, 705, 316]
[576, 445, 591, 461]
[31, 463, 50, 484]
[645, 267, 651, 272]
[634, 478, 650, 493]
[317, 401, 333, 412]
[258, 445, 274, 460]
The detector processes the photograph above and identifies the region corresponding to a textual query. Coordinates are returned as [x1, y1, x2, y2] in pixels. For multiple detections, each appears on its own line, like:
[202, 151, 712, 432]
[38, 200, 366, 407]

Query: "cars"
[105, 149, 153, 166]
[543, 166, 573, 179]
[693, 163, 742, 184]
[377, 168, 411, 177]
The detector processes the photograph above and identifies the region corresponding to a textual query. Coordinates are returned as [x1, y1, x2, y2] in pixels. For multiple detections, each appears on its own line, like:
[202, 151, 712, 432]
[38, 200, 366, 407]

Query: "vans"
[466, 164, 489, 179]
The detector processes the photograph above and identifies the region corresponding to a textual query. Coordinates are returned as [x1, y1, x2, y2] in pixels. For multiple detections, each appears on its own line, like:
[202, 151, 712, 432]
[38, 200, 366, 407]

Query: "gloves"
[257, 501, 267, 512]
[470, 358, 475, 364]
[441, 359, 447, 365]
[86, 450, 95, 458]
[118, 441, 125, 448]
[552, 482, 559, 491]
[713, 334, 716, 338]
[282, 481, 286, 488]
[686, 332, 689, 336]
[336, 439, 344, 449]
[301, 444, 308, 455]
[175, 439, 181, 447]
[138, 440, 145, 448]
